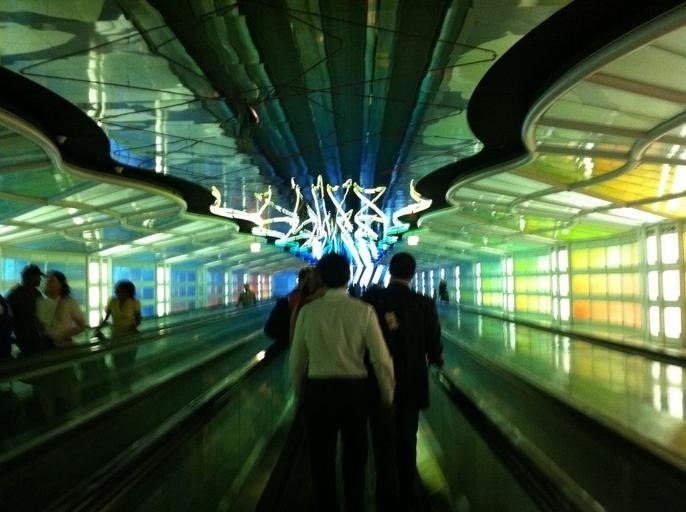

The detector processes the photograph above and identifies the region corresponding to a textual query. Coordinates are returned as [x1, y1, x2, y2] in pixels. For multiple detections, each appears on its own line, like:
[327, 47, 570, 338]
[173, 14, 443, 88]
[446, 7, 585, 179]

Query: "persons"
[365, 252, 443, 506]
[3, 261, 75, 413]
[0, 296, 14, 366]
[287, 249, 398, 511]
[31, 270, 87, 414]
[233, 283, 257, 307]
[93, 280, 141, 397]
[263, 268, 450, 362]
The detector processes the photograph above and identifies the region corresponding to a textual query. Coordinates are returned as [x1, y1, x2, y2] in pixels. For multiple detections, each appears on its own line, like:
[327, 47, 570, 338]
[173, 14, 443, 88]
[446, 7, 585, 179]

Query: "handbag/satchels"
[264, 297, 291, 343]
[17, 336, 55, 385]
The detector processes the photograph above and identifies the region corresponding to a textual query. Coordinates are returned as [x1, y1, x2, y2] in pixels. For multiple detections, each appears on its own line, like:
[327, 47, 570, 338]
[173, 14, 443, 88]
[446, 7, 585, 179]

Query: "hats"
[21, 264, 48, 278]
